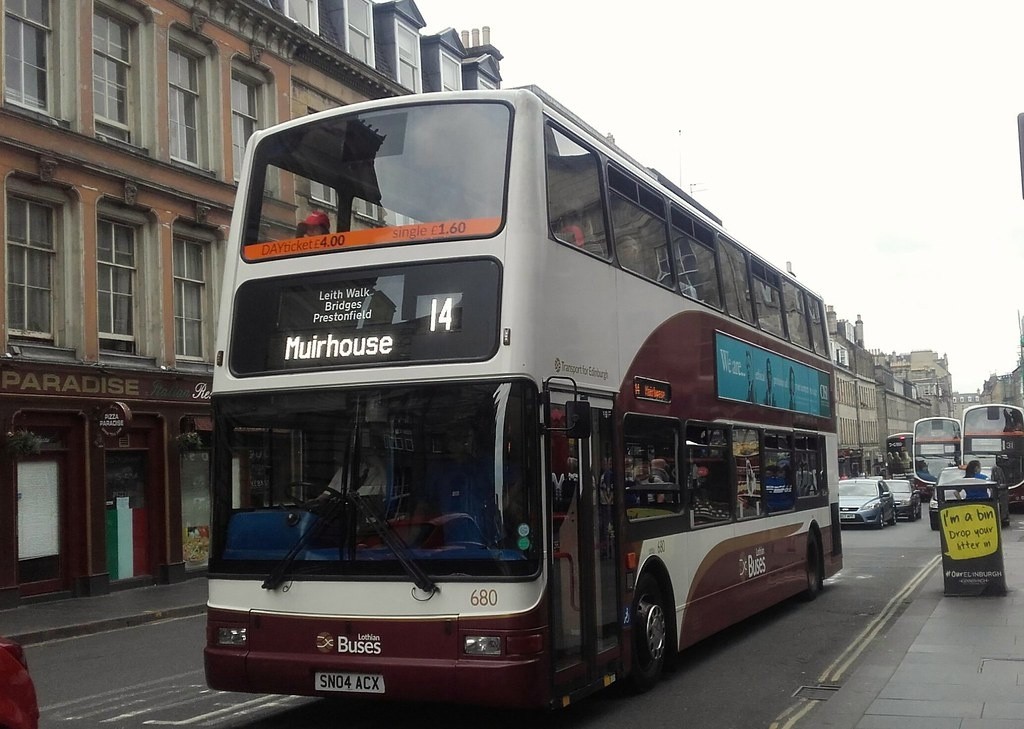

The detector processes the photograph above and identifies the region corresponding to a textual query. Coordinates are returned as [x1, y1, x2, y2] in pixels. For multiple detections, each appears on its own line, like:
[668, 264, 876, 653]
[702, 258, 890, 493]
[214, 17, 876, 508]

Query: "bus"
[887, 405, 1024, 508]
[203, 84, 846, 718]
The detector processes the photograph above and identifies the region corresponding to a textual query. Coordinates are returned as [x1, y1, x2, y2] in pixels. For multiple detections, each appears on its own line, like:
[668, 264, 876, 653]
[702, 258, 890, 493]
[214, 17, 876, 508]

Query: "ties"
[357, 469, 369, 489]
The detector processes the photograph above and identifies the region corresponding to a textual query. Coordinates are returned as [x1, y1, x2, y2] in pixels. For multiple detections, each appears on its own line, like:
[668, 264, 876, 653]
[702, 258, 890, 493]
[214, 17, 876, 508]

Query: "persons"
[953, 459, 995, 501]
[561, 224, 640, 275]
[296, 209, 331, 238]
[744, 351, 797, 410]
[425, 435, 669, 547]
[305, 438, 389, 498]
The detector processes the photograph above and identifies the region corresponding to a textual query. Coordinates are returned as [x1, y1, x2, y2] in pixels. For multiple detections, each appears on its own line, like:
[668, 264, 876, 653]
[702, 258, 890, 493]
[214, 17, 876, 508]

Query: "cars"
[837, 477, 897, 530]
[884, 481, 923, 522]
[0, 639, 40, 728]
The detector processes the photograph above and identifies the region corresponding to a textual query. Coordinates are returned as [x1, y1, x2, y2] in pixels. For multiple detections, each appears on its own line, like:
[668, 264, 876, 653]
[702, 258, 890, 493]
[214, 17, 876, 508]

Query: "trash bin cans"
[936, 478, 1010, 598]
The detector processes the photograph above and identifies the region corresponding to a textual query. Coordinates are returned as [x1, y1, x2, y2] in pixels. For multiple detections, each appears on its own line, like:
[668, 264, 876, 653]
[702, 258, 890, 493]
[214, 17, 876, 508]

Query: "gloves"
[295, 225, 306, 238]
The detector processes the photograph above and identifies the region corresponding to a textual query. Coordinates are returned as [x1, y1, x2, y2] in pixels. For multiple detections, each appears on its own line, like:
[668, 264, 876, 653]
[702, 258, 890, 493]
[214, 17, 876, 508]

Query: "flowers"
[175, 431, 203, 459]
[8, 428, 42, 458]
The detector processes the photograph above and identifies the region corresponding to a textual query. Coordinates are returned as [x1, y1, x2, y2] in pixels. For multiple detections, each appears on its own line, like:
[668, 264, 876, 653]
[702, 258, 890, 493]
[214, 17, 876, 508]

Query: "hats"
[299, 211, 330, 228]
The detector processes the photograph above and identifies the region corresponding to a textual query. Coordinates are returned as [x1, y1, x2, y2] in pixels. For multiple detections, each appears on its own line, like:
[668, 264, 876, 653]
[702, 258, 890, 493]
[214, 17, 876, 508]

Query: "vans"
[928, 467, 1010, 531]
